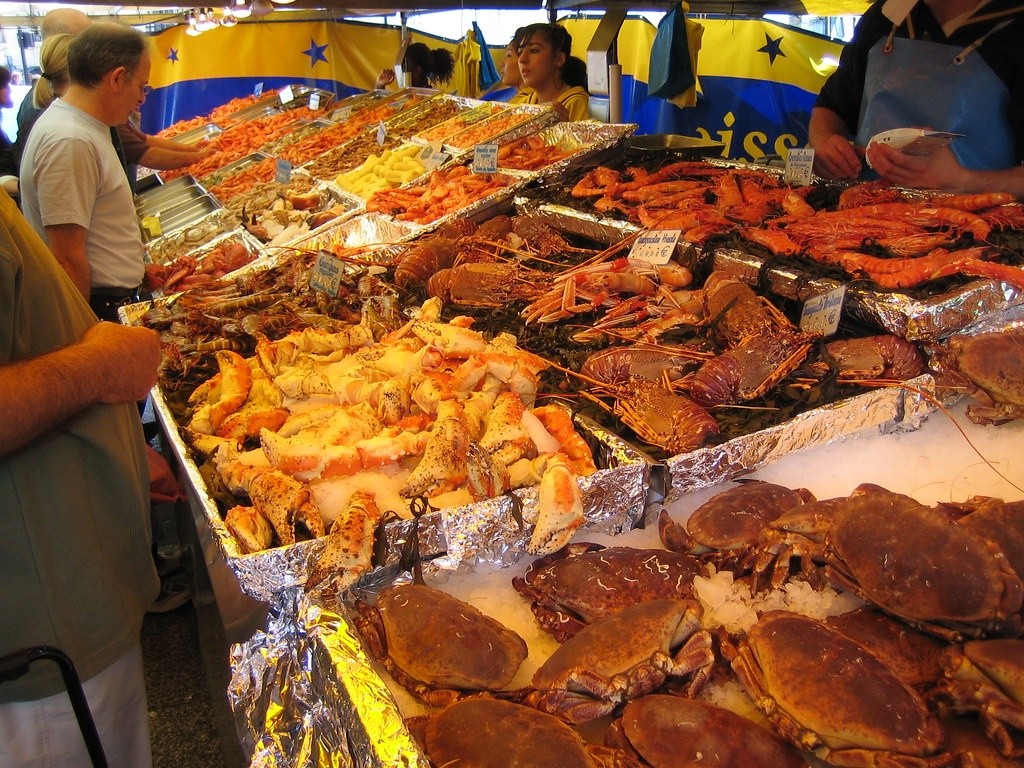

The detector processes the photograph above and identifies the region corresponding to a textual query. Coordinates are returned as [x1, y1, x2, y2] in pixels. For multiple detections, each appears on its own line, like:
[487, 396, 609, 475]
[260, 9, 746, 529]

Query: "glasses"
[128, 69, 153, 95]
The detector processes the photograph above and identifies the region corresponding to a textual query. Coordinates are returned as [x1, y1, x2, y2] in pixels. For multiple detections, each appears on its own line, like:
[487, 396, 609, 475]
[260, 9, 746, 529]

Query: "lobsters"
[384, 208, 925, 456]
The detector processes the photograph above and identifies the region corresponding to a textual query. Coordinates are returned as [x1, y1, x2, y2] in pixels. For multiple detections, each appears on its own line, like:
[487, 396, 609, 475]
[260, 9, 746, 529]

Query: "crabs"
[924, 325, 1024, 429]
[353, 477, 1024, 768]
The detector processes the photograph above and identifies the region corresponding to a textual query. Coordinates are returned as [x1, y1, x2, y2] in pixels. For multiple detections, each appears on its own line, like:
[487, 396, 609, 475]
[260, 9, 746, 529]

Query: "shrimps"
[149, 174, 316, 267]
[129, 251, 420, 396]
[570, 158, 1024, 289]
[497, 136, 573, 170]
[366, 166, 523, 224]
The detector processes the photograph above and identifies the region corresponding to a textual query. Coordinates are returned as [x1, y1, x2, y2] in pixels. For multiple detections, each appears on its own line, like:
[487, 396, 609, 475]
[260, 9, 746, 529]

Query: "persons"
[805, 0, 1024, 196]
[375, 42, 455, 91]
[499, 22, 590, 122]
[0, 184, 164, 768]
[0, 7, 218, 613]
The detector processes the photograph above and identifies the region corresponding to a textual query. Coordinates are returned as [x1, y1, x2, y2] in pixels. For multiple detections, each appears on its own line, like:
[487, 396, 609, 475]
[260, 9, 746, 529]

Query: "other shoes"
[146, 577, 192, 613]
[156, 555, 180, 576]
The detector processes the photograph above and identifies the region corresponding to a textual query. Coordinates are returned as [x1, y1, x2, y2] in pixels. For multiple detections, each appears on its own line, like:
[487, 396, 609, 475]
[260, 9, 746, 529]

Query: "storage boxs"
[117, 83, 1024, 604]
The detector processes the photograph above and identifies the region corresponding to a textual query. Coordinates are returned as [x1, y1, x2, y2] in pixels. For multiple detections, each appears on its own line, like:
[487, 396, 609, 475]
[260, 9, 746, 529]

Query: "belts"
[91, 285, 141, 296]
